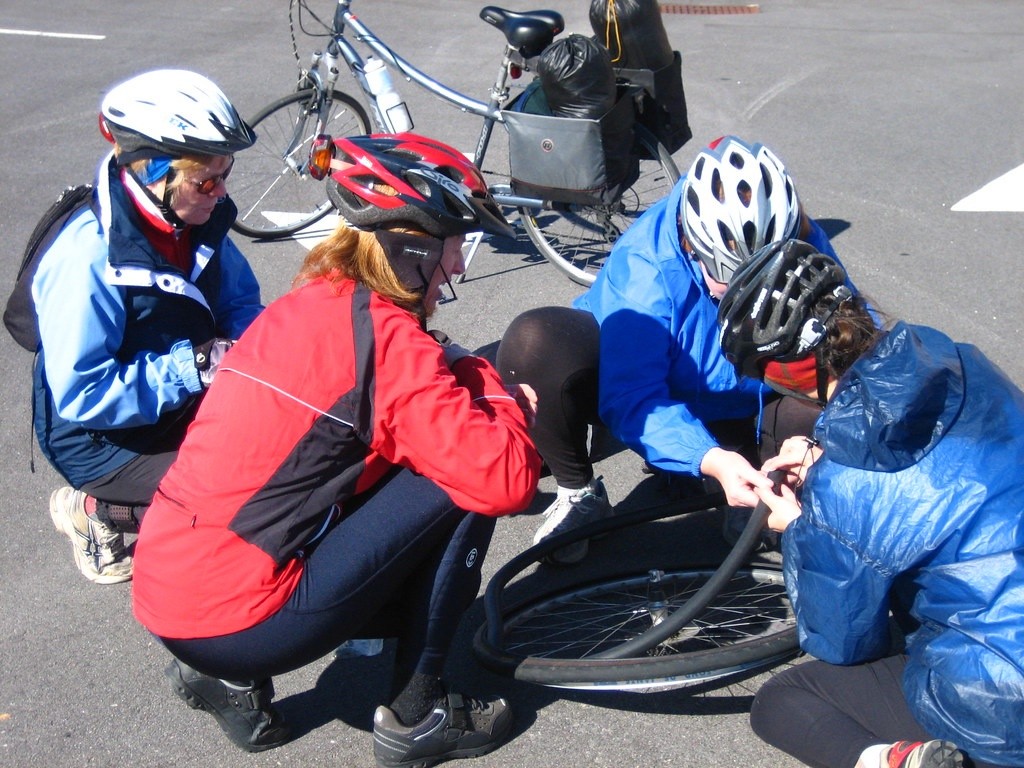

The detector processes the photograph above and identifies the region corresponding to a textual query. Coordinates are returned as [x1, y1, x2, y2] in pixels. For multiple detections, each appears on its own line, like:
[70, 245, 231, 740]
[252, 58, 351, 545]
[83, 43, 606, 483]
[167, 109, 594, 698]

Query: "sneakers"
[49, 487, 134, 585]
[533, 479, 615, 566]
[374, 690, 514, 768]
[722, 506, 777, 553]
[163, 658, 291, 751]
[880, 739, 963, 768]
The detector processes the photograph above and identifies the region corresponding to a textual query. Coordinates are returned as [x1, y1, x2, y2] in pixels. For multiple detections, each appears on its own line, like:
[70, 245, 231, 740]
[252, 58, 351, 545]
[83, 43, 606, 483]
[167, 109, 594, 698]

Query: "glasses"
[186, 155, 235, 193]
[437, 261, 458, 305]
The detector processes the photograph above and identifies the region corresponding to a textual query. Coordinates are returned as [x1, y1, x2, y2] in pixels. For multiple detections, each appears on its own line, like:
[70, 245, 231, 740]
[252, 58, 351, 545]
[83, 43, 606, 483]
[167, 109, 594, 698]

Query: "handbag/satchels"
[612, 51, 693, 163]
[499, 85, 642, 205]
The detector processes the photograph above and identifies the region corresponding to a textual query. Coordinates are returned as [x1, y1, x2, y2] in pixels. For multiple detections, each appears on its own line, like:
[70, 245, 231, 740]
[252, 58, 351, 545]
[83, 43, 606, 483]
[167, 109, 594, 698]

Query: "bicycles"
[213, 0, 688, 289]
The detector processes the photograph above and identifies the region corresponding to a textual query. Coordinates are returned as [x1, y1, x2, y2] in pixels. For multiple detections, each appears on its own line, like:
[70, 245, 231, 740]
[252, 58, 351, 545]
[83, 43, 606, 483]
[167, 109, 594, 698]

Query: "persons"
[498, 135, 881, 571]
[31, 68, 267, 585]
[711, 238, 1023, 768]
[133, 132, 540, 768]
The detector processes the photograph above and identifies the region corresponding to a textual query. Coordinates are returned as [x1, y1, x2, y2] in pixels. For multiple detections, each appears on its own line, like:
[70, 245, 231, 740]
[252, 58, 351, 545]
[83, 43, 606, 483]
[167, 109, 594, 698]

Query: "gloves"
[193, 338, 238, 389]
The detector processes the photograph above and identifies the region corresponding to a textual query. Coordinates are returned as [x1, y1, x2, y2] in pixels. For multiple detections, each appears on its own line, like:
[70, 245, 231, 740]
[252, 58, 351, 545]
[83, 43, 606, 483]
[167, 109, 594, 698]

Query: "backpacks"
[2, 184, 93, 351]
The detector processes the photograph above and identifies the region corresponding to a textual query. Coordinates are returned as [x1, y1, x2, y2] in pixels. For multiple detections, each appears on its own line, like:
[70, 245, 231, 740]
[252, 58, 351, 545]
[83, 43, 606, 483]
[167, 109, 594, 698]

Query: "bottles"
[363, 55, 415, 139]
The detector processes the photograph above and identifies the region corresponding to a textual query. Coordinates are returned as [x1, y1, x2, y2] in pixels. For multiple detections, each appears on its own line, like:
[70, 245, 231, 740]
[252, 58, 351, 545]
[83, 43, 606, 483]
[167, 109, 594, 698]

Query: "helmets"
[325, 134, 519, 241]
[717, 240, 855, 378]
[101, 69, 258, 156]
[681, 134, 801, 283]
